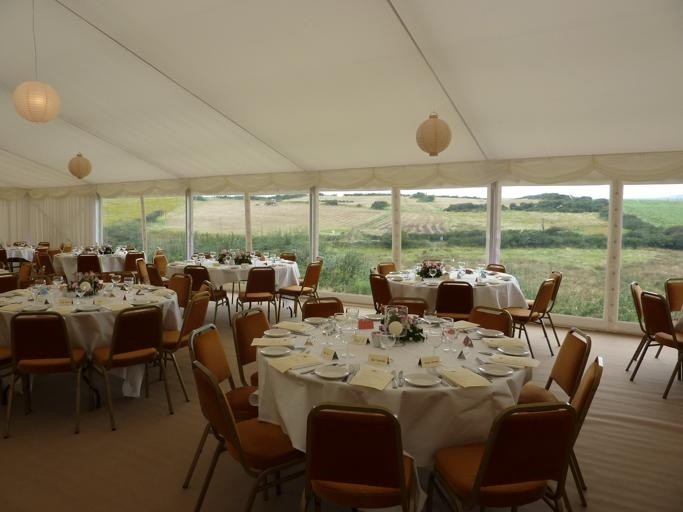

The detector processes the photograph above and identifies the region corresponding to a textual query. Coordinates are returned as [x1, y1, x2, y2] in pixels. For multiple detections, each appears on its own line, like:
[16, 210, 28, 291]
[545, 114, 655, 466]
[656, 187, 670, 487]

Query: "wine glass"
[192, 249, 296, 267]
[368, 306, 408, 367]
[321, 308, 359, 346]
[390, 258, 512, 287]
[75, 239, 106, 256]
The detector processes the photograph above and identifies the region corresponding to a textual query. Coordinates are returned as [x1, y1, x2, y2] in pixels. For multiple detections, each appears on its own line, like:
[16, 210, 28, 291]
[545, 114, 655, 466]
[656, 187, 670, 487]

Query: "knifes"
[299, 363, 338, 374]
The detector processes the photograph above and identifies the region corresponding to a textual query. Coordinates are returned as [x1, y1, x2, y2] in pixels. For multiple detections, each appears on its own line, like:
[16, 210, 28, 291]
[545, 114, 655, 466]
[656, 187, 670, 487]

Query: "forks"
[390, 370, 403, 389]
[341, 367, 358, 384]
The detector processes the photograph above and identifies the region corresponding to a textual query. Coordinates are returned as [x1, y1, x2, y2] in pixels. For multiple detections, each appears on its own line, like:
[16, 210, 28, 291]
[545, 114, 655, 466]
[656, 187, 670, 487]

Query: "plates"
[363, 313, 384, 321]
[404, 309, 532, 387]
[313, 366, 350, 380]
[20, 274, 176, 313]
[261, 317, 327, 357]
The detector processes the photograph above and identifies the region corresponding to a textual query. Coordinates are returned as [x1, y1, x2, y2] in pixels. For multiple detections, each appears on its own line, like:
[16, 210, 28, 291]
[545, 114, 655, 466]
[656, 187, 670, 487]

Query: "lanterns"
[12, 80, 62, 121]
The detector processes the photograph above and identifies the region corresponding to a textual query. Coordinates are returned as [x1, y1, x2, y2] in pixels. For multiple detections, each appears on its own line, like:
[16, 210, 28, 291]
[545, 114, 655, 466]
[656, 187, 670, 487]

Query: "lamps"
[12, 1, 61, 123]
